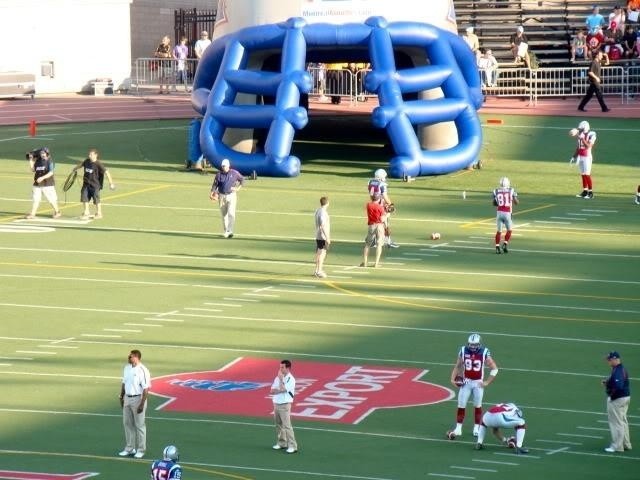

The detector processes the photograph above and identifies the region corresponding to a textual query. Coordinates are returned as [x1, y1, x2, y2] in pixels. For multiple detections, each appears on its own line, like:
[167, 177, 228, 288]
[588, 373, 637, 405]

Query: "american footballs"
[455, 376, 464, 386]
[507, 436, 516, 449]
[431, 232, 440, 240]
[212, 192, 219, 201]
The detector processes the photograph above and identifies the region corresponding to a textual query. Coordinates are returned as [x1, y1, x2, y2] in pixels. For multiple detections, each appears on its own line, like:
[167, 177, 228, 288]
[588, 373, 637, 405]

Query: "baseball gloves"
[569, 129, 580, 138]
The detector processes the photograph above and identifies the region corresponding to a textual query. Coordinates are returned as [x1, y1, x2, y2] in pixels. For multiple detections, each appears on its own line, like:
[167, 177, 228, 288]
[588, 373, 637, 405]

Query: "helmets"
[499, 177, 509, 188]
[577, 121, 590, 134]
[468, 334, 481, 347]
[375, 169, 387, 179]
[162, 445, 179, 462]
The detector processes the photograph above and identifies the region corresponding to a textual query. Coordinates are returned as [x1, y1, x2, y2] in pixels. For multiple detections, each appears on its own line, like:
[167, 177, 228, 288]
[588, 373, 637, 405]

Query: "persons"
[25, 145, 116, 220]
[634, 184, 640, 204]
[567, 120, 598, 199]
[570, 1, 639, 62]
[206, 158, 246, 238]
[268, 359, 300, 453]
[118, 350, 151, 458]
[463, 27, 497, 86]
[150, 445, 183, 480]
[314, 169, 394, 279]
[450, 332, 530, 455]
[601, 350, 633, 452]
[577, 50, 611, 112]
[490, 177, 519, 253]
[195, 31, 211, 60]
[155, 35, 192, 93]
[510, 26, 527, 62]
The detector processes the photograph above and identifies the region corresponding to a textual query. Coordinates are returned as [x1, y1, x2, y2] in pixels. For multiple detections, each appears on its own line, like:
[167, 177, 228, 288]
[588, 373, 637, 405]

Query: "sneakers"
[386, 242, 399, 249]
[272, 445, 285, 449]
[495, 191, 639, 254]
[119, 449, 136, 456]
[134, 450, 144, 457]
[452, 429, 530, 453]
[286, 448, 297, 453]
[605, 447, 623, 452]
[24, 210, 102, 219]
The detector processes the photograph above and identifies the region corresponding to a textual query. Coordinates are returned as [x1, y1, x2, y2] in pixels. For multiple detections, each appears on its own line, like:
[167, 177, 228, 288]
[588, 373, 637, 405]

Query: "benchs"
[453, 0, 640, 99]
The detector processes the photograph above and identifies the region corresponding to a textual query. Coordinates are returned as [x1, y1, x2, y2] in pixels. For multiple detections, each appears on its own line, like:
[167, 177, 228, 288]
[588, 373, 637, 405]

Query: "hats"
[607, 352, 620, 359]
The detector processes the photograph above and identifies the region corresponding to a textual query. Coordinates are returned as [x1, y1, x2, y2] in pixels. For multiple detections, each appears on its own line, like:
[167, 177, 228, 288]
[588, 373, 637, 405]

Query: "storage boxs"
[91, 82, 114, 96]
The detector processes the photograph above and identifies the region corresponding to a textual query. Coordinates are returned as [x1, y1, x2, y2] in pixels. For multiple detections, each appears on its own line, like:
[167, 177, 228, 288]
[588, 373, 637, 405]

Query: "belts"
[128, 395, 140, 397]
